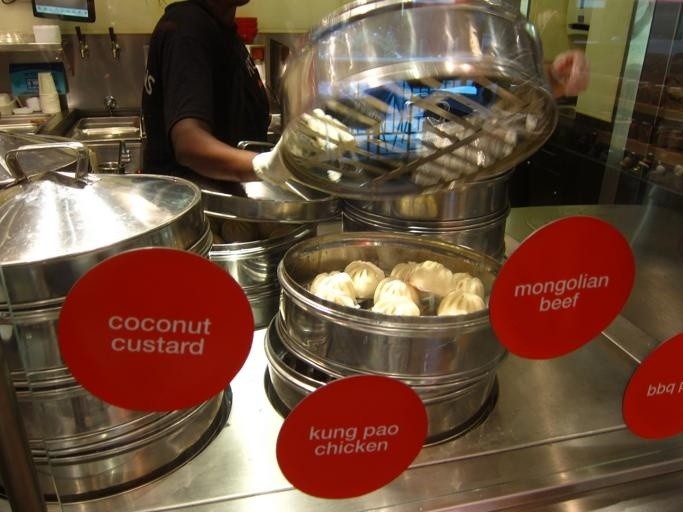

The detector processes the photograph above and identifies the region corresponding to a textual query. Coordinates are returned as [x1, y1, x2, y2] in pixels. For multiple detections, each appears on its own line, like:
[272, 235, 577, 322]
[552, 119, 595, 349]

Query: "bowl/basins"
[33, 25, 61, 44]
[0, 32, 27, 44]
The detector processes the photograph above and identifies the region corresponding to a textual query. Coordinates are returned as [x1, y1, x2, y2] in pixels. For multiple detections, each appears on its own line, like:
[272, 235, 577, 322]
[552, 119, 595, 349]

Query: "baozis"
[284, 109, 354, 182]
[309, 260, 491, 316]
[411, 109, 538, 186]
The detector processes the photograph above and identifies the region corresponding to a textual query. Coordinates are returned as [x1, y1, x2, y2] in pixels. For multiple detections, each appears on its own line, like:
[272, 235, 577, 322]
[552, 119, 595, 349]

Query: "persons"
[551, 43, 589, 107]
[141, 1, 358, 200]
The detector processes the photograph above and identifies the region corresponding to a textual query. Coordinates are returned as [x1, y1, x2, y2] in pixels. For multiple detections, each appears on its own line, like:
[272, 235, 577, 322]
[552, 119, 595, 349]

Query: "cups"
[26, 98, 40, 112]
[37, 73, 60, 114]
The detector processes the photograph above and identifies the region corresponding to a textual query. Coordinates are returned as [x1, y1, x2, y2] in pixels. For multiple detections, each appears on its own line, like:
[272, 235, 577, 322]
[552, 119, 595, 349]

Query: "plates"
[12, 109, 32, 115]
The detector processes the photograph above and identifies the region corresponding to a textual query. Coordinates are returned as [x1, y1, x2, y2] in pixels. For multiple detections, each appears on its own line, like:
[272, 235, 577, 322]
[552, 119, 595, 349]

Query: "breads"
[382, 196, 440, 220]
